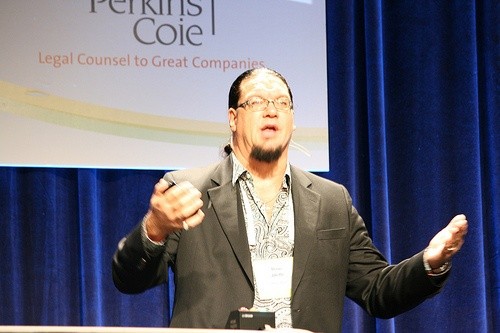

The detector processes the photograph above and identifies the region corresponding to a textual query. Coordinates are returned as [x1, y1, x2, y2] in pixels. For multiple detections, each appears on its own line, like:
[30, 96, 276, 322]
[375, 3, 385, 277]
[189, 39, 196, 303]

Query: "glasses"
[234, 96, 295, 113]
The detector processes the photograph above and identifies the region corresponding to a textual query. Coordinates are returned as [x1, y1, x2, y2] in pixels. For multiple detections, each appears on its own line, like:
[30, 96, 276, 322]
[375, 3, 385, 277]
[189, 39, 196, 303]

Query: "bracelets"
[422, 247, 448, 273]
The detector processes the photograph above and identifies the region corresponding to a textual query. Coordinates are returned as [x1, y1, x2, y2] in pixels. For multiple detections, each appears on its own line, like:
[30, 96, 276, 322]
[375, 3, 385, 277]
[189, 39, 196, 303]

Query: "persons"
[112, 67, 468, 333]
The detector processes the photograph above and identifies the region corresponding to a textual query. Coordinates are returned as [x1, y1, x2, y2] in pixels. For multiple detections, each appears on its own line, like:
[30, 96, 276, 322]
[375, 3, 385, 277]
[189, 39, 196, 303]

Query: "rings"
[182, 220, 189, 232]
[447, 247, 457, 250]
